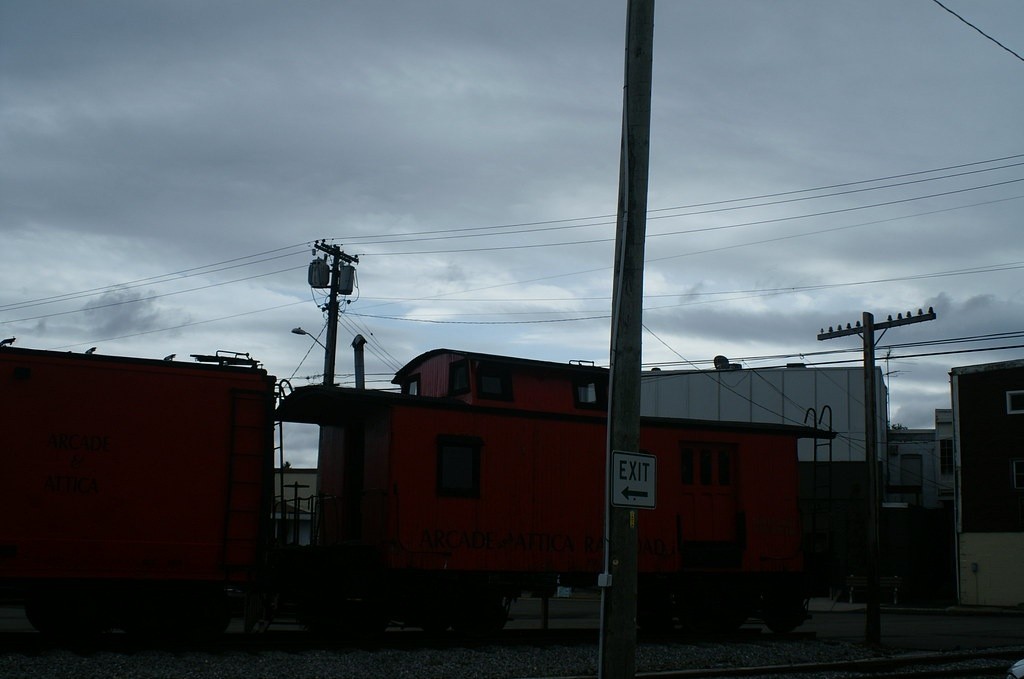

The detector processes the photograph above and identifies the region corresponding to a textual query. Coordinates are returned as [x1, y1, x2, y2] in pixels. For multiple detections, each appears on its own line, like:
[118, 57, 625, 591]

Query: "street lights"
[290, 327, 336, 384]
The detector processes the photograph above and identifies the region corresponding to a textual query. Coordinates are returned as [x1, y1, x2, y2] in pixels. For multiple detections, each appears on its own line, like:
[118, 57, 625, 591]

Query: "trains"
[0, 338, 842, 640]
